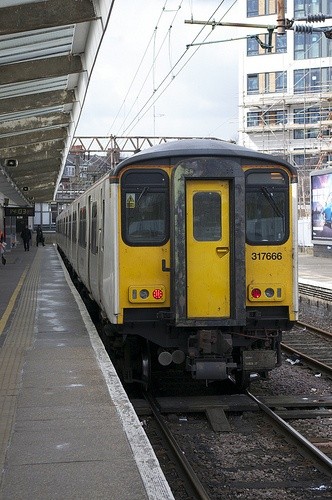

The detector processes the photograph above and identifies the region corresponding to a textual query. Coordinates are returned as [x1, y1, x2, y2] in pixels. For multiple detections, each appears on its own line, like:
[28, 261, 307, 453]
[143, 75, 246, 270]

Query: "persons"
[35, 225, 46, 247]
[20, 225, 31, 251]
[320, 202, 332, 228]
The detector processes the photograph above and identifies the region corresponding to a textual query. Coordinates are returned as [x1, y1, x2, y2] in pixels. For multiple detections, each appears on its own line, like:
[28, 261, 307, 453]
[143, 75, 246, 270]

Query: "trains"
[56, 137, 302, 399]
